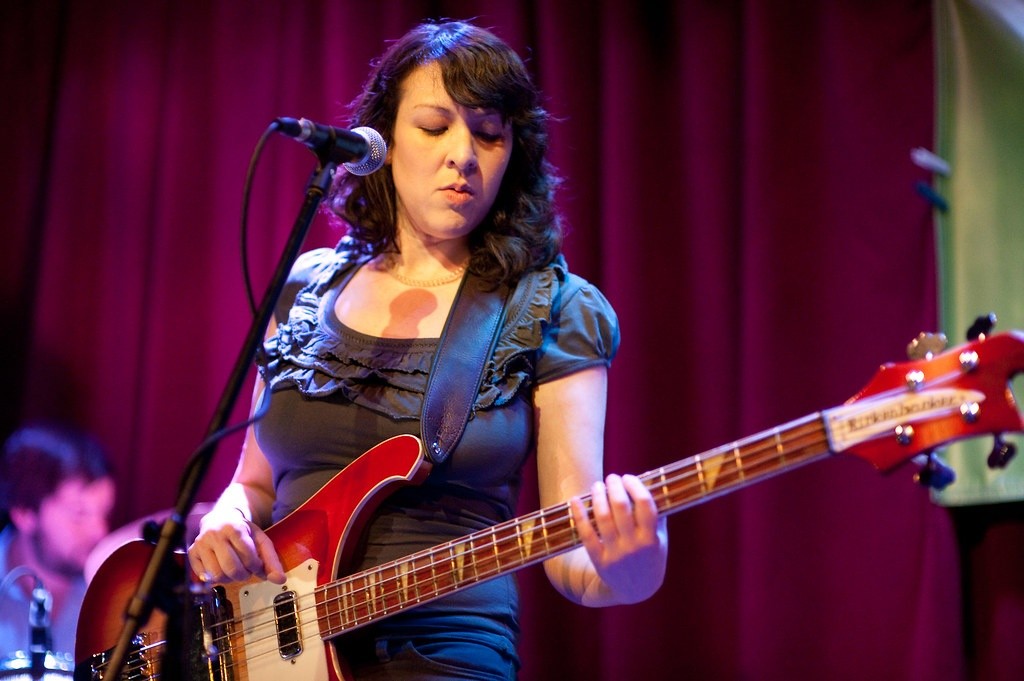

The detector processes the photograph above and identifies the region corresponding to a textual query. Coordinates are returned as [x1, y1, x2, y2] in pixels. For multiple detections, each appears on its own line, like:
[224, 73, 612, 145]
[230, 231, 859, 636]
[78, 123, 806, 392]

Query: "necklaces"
[377, 256, 471, 288]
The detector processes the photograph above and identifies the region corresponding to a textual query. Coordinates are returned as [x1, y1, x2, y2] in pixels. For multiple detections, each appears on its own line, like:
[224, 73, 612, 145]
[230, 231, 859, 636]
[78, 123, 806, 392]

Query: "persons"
[185, 25, 670, 681]
[0, 420, 116, 681]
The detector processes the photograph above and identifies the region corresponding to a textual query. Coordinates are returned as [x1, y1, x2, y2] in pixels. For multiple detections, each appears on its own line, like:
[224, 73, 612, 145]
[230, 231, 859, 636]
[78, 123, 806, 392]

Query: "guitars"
[72, 313, 1024, 681]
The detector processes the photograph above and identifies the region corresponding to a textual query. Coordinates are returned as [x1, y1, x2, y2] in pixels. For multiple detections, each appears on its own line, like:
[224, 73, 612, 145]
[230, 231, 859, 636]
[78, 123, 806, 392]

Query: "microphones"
[27, 588, 51, 681]
[274, 117, 387, 177]
